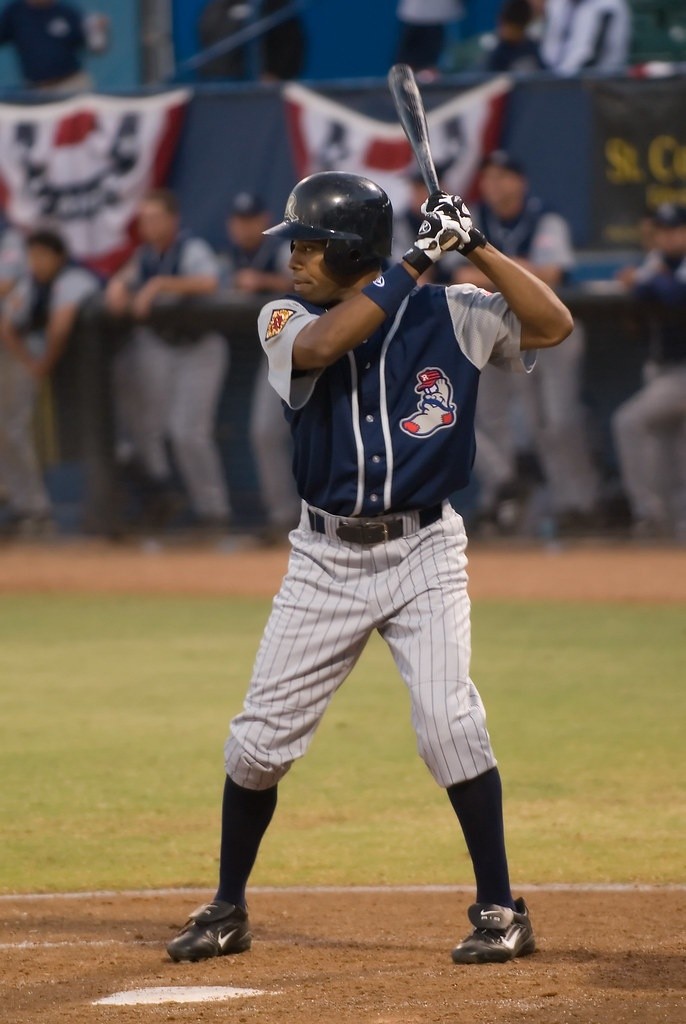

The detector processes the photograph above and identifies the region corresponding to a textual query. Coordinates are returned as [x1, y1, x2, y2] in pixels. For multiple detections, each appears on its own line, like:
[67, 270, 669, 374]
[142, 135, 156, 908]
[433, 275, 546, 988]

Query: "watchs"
[457, 227, 487, 256]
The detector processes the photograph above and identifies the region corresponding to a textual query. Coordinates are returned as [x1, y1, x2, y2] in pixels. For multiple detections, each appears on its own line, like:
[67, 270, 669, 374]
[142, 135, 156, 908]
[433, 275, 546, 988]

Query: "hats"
[231, 193, 262, 213]
[477, 147, 523, 177]
[656, 203, 686, 225]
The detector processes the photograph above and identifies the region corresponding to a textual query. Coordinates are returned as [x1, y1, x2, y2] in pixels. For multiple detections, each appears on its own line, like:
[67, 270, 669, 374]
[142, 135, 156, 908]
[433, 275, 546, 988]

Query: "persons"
[0, 0, 686, 542]
[163, 171, 574, 962]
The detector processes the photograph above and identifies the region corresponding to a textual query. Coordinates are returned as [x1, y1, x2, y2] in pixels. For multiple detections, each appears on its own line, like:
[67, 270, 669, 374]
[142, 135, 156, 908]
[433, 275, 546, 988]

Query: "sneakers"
[452, 897, 535, 964]
[167, 900, 252, 961]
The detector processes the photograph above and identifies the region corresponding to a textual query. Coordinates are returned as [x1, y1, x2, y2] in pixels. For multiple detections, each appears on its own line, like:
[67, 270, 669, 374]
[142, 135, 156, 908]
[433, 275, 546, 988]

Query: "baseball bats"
[387, 61, 460, 254]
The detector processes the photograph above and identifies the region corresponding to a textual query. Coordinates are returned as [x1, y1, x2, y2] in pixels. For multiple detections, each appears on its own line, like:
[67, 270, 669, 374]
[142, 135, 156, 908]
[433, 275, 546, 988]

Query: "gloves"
[402, 202, 470, 275]
[420, 190, 487, 256]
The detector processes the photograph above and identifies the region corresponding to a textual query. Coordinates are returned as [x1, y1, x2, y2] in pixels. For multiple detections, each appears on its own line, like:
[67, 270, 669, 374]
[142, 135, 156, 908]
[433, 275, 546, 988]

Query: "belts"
[308, 502, 442, 545]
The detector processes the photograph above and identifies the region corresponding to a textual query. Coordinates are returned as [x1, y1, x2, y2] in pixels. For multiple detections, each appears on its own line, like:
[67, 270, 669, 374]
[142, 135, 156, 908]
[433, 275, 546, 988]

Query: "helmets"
[262, 171, 393, 277]
